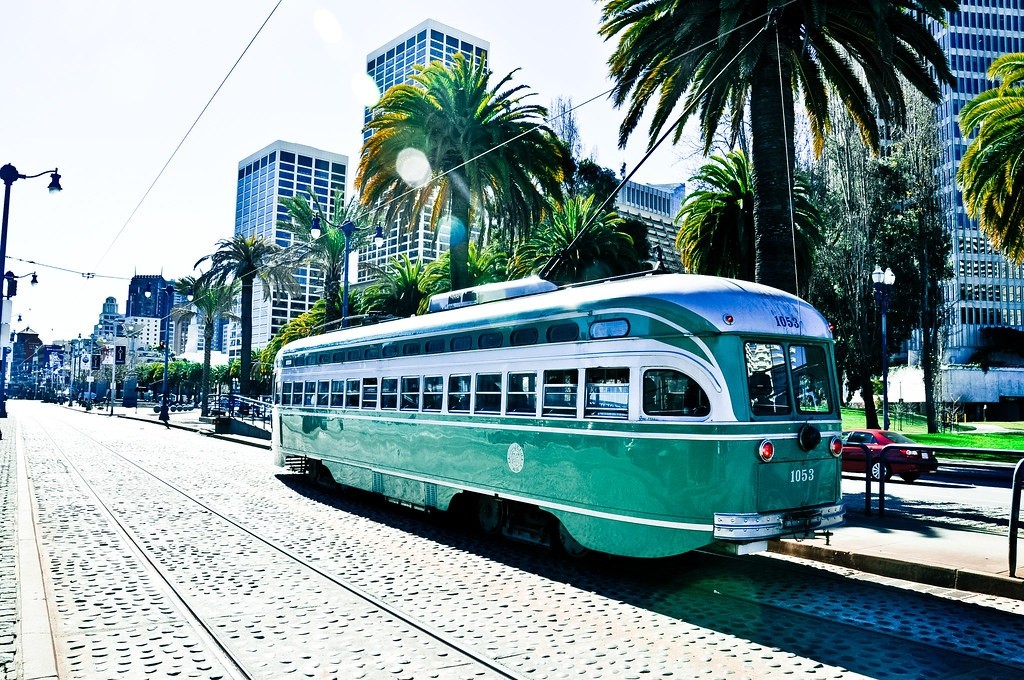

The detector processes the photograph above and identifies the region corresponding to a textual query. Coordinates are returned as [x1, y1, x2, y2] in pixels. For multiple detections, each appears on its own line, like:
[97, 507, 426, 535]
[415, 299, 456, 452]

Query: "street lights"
[98, 318, 133, 414]
[76, 333, 82, 404]
[314, 213, 383, 328]
[871, 264, 896, 431]
[68, 345, 76, 406]
[0, 162, 63, 441]
[86, 334, 96, 410]
[145, 281, 194, 420]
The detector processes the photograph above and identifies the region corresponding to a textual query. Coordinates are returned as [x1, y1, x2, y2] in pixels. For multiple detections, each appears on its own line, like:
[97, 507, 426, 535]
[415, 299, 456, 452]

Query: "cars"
[841, 428, 939, 483]
[78, 392, 97, 405]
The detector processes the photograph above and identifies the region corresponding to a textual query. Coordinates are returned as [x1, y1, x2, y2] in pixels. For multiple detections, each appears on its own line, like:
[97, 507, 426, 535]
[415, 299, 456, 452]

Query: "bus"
[271, 275, 846, 559]
